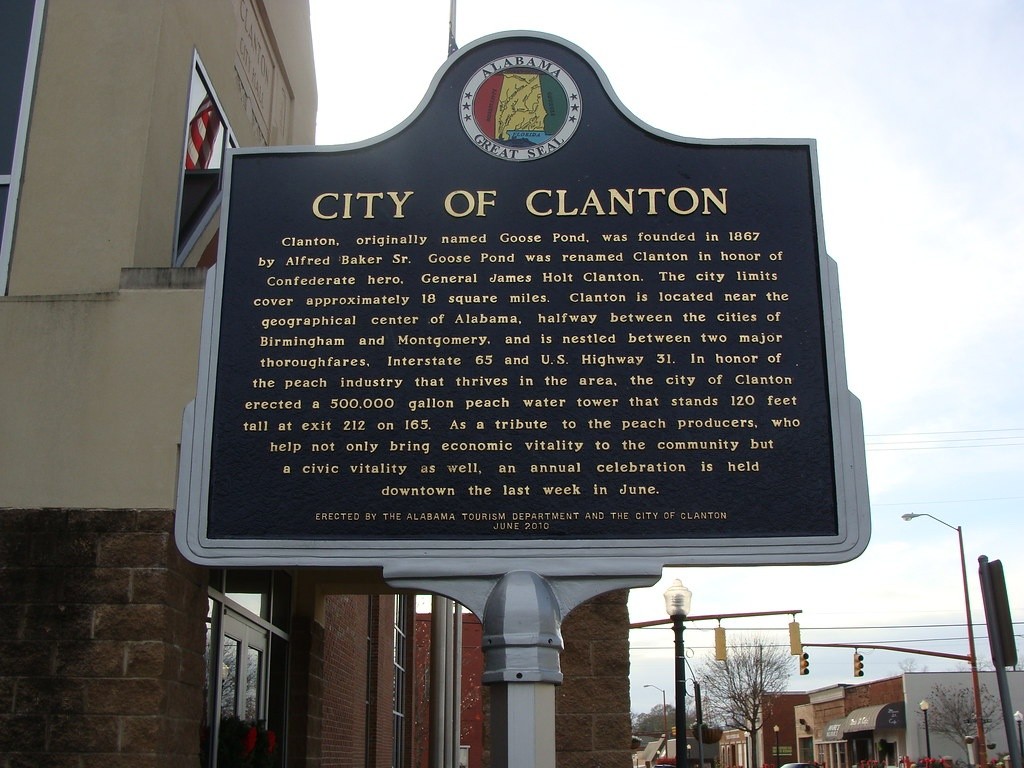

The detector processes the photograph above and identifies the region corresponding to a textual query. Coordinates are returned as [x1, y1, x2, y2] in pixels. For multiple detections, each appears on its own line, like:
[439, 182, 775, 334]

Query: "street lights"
[744, 731, 749, 768]
[687, 744, 691, 758]
[1014, 710, 1024, 760]
[663, 579, 694, 756]
[657, 750, 660, 759]
[920, 700, 931, 759]
[774, 725, 780, 767]
[644, 684, 668, 758]
[901, 513, 989, 768]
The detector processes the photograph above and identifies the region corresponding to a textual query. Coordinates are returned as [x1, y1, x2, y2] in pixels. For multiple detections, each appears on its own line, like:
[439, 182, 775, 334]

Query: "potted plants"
[630, 736, 642, 749]
[691, 721, 724, 744]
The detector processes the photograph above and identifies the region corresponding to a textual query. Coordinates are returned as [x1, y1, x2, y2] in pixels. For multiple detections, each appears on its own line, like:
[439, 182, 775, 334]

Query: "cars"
[780, 763, 820, 768]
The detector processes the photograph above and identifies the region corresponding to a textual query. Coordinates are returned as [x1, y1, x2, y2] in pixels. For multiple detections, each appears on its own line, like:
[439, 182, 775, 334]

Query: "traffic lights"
[854, 654, 864, 677]
[800, 652, 809, 675]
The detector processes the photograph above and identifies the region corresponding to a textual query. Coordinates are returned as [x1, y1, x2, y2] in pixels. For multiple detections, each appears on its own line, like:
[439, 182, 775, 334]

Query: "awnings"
[822, 718, 846, 741]
[843, 702, 906, 732]
